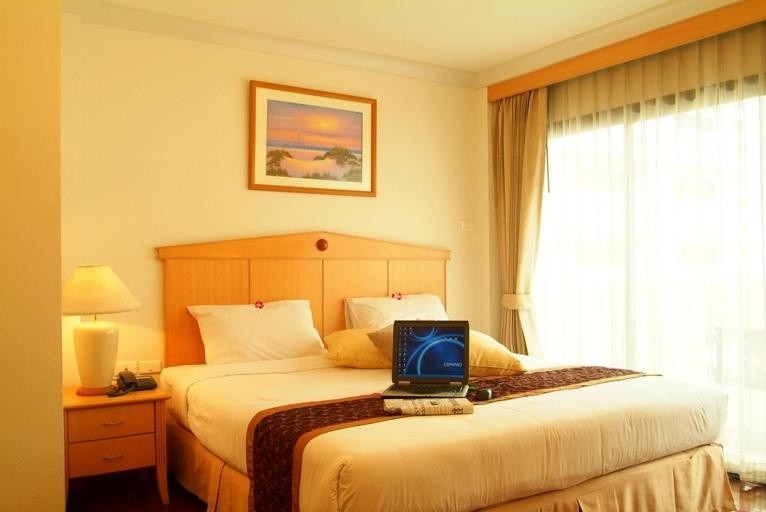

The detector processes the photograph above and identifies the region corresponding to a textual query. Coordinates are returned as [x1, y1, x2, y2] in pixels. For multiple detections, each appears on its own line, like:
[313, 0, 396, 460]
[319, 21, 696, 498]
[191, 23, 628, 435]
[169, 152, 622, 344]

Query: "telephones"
[117, 371, 157, 391]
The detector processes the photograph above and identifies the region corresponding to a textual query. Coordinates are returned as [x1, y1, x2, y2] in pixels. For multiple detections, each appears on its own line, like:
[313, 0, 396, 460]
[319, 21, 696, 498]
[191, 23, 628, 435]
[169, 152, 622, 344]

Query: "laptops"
[381, 320, 469, 398]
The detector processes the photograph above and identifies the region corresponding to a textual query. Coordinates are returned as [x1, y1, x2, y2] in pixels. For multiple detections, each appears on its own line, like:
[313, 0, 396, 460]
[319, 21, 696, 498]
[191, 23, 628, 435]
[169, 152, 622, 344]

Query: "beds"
[155, 230, 738, 511]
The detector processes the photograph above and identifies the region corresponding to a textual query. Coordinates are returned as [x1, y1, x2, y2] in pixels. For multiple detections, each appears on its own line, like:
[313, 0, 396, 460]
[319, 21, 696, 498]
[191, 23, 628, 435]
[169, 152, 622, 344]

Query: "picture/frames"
[247, 78, 377, 198]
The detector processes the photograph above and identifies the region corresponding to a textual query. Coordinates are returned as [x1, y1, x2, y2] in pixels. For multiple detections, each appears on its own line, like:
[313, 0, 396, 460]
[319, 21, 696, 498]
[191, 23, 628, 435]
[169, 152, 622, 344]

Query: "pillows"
[185, 298, 328, 367]
[343, 296, 449, 330]
[322, 327, 391, 370]
[366, 322, 528, 377]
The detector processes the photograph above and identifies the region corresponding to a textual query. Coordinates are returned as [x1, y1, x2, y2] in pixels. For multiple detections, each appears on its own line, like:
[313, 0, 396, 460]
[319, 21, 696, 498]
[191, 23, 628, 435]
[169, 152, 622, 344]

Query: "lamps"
[60, 263, 141, 397]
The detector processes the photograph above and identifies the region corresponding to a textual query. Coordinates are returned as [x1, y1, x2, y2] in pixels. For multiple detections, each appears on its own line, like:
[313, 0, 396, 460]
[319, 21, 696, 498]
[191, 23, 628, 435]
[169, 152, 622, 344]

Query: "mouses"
[476, 389, 491, 400]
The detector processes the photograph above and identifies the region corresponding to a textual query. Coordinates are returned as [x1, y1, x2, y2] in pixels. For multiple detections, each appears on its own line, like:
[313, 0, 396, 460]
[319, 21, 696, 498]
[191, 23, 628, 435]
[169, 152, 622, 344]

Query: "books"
[383, 397, 474, 416]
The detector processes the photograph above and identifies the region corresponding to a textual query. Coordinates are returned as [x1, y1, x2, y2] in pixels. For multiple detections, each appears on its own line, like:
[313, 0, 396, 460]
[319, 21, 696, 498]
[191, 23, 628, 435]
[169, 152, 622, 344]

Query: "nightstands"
[60, 374, 174, 505]
